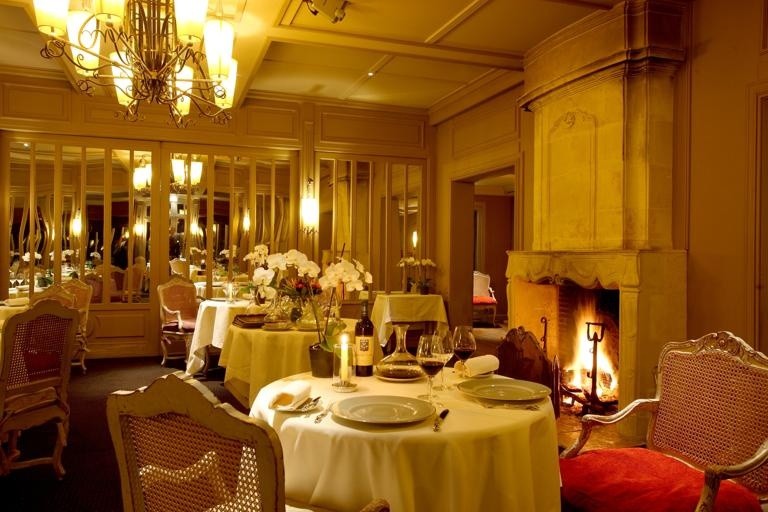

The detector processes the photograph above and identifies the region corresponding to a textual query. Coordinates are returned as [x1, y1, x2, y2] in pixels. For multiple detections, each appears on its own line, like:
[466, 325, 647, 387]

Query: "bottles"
[355, 302, 375, 378]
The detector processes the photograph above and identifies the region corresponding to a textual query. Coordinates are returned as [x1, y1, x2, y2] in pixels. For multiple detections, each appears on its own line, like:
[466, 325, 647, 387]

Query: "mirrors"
[86, 147, 104, 304]
[61, 144, 81, 283]
[133, 150, 152, 304]
[34, 143, 55, 295]
[190, 154, 208, 298]
[274, 160, 290, 254]
[373, 161, 385, 294]
[319, 160, 334, 277]
[110, 149, 130, 303]
[169, 153, 189, 283]
[212, 155, 230, 299]
[392, 163, 404, 293]
[8, 142, 31, 299]
[256, 159, 271, 255]
[234, 155, 250, 295]
[337, 161, 352, 264]
[407, 165, 420, 293]
[356, 161, 370, 273]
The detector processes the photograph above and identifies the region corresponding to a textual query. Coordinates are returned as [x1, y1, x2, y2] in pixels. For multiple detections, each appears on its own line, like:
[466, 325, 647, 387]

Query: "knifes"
[433, 408, 450, 432]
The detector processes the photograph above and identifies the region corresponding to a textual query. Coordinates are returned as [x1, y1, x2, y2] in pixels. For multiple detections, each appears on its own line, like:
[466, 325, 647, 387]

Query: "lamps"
[34, 0, 238, 128]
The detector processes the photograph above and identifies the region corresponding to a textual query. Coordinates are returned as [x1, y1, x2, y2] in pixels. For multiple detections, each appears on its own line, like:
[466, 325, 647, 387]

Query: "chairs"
[169, 258, 194, 284]
[86, 273, 104, 303]
[111, 267, 128, 303]
[106, 371, 391, 512]
[0, 299, 81, 480]
[29, 285, 78, 309]
[473, 271, 498, 327]
[559, 330, 768, 512]
[133, 264, 143, 303]
[58, 279, 93, 371]
[156, 277, 198, 366]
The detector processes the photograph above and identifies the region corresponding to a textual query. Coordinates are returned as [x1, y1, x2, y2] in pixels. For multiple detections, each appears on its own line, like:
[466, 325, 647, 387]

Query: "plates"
[261, 327, 294, 331]
[474, 370, 496, 378]
[330, 395, 436, 425]
[372, 374, 427, 383]
[268, 392, 321, 413]
[3, 297, 31, 307]
[212, 281, 224, 287]
[296, 328, 325, 332]
[457, 378, 552, 401]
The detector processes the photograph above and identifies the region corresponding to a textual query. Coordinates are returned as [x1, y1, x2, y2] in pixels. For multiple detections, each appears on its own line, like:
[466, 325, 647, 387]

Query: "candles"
[229, 283, 233, 300]
[340, 334, 349, 383]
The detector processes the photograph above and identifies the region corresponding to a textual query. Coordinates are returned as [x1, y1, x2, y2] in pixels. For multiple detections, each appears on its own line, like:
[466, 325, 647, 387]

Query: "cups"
[225, 284, 236, 304]
[331, 344, 358, 392]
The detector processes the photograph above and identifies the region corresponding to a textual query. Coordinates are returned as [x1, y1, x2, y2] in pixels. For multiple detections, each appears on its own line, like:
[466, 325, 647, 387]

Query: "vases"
[420, 285, 429, 294]
[309, 342, 342, 378]
[407, 279, 415, 292]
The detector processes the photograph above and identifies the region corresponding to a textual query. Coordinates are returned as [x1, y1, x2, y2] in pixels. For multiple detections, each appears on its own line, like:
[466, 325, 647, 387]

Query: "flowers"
[396, 257, 420, 279]
[421, 258, 436, 285]
[298, 249, 373, 342]
[253, 249, 298, 300]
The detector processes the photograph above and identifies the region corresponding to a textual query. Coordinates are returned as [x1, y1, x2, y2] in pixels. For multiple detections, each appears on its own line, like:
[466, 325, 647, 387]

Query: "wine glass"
[416, 335, 446, 404]
[451, 325, 476, 383]
[431, 330, 454, 392]
